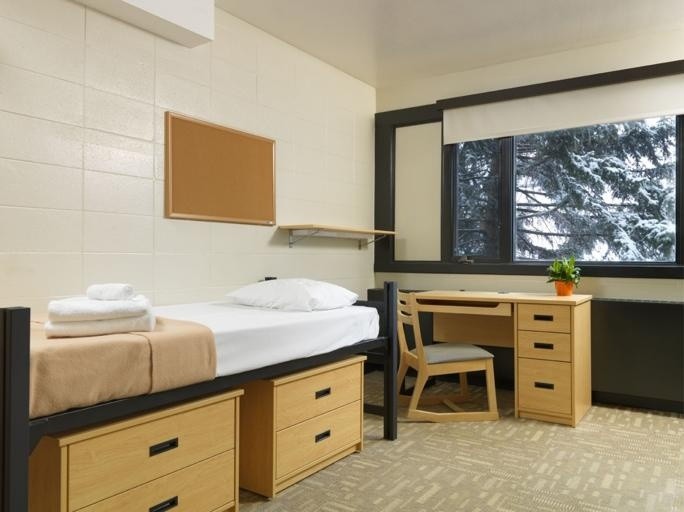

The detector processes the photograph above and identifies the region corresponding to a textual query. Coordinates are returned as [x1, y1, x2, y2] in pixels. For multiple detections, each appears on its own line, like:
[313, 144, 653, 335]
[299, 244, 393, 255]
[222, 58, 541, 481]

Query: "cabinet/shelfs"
[28, 388, 244, 512]
[240, 354, 368, 499]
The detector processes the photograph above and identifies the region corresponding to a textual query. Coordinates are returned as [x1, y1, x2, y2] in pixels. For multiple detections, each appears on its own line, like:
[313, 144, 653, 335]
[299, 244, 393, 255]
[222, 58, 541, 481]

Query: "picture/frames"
[165, 109, 277, 228]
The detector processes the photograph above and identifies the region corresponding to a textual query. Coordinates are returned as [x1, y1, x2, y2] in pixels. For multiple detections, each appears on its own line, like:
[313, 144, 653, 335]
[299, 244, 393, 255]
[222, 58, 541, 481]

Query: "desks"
[411, 289, 592, 428]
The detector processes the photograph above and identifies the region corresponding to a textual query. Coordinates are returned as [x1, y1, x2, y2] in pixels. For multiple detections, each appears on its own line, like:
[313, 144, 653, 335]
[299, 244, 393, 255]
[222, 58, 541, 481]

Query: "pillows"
[226, 277, 359, 311]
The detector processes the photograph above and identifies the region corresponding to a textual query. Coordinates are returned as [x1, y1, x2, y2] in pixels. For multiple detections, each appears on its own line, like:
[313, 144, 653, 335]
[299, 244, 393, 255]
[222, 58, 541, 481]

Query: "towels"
[86, 282, 137, 300]
[44, 305, 157, 337]
[48, 293, 146, 322]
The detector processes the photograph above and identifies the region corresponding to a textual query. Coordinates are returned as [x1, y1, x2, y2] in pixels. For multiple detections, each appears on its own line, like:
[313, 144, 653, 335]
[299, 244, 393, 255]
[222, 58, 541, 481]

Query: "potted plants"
[546, 255, 581, 296]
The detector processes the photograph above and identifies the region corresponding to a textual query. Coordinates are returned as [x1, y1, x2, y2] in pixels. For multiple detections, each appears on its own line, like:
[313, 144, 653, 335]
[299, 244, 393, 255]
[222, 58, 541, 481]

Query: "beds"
[1, 277, 399, 511]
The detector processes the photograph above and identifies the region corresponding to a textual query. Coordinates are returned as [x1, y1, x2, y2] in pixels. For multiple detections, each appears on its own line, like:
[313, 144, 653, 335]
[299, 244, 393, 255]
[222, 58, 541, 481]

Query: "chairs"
[396, 287, 500, 422]
